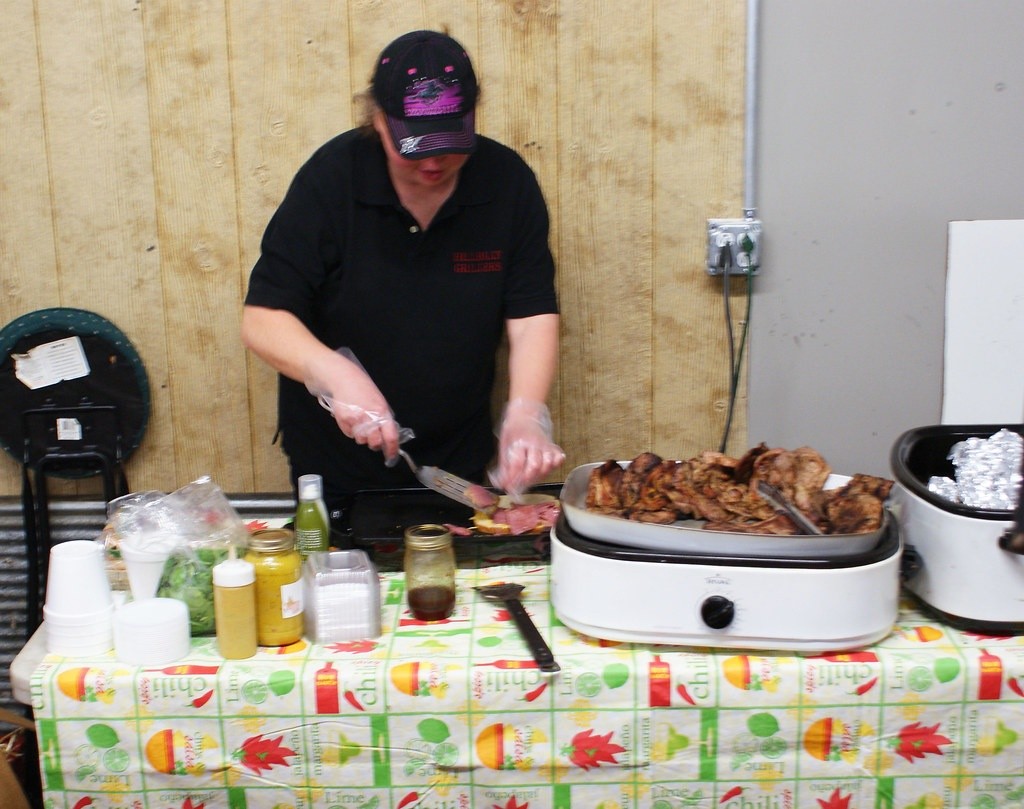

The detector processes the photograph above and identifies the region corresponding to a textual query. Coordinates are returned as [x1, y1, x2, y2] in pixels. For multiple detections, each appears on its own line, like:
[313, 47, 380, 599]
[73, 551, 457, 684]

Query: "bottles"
[212, 548, 258, 661]
[243, 528, 306, 649]
[294, 473, 330, 561]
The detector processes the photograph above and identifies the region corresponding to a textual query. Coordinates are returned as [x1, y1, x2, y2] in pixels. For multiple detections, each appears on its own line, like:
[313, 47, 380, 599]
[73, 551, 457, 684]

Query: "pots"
[550, 511, 922, 652]
[889, 424, 1024, 635]
[334, 482, 563, 568]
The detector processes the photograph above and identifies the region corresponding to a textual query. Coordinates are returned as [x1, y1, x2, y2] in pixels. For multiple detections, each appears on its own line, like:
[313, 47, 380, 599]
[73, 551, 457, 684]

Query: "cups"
[404, 525, 458, 623]
[120, 544, 169, 602]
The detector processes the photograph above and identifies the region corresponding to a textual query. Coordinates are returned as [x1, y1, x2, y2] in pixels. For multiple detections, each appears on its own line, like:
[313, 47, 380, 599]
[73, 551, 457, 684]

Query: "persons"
[233, 30, 572, 569]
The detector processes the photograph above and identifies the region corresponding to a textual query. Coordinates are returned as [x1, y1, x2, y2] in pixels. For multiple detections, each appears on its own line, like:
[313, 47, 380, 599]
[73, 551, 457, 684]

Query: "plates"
[560, 460, 888, 558]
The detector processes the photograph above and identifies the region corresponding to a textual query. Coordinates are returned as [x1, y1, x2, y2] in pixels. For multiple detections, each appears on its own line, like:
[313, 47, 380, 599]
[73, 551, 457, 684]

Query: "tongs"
[756, 478, 878, 535]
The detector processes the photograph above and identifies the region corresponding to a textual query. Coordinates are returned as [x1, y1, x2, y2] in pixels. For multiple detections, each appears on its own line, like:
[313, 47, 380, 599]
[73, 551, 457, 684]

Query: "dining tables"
[8, 563, 1024, 809]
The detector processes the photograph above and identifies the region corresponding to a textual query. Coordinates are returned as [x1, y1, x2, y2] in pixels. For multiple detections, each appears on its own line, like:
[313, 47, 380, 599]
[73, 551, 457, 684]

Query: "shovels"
[316, 394, 501, 517]
[472, 583, 563, 672]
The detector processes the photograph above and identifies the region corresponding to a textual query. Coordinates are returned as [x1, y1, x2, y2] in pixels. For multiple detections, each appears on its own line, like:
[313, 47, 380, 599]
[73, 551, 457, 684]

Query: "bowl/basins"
[44, 538, 117, 658]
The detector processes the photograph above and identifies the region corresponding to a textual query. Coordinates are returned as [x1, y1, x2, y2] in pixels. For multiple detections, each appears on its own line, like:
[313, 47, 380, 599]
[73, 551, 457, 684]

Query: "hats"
[371, 30, 478, 161]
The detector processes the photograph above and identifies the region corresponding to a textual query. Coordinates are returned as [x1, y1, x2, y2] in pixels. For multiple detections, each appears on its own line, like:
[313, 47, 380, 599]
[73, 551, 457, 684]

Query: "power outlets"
[706, 216, 762, 278]
